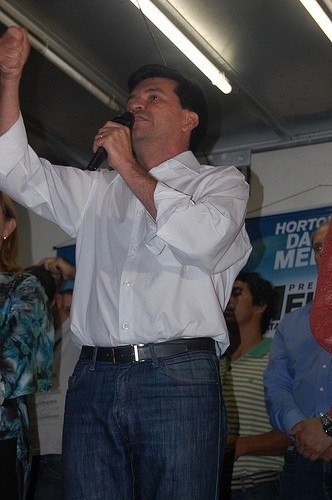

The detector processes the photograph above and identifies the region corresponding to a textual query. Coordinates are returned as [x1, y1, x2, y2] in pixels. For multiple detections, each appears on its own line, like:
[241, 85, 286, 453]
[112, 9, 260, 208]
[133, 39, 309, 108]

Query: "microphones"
[87, 111, 134, 171]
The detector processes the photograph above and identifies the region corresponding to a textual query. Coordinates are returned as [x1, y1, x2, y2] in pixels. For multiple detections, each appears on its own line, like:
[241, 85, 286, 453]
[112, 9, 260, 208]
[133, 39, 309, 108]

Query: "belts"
[231, 471, 279, 489]
[80, 337, 216, 364]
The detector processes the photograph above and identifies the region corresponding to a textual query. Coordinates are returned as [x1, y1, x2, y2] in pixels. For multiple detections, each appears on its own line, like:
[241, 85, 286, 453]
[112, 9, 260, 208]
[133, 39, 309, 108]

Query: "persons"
[14, 255, 84, 500]
[0, 190, 61, 500]
[261, 220, 332, 500]
[0, 23, 255, 500]
[310, 213, 332, 354]
[218, 270, 299, 500]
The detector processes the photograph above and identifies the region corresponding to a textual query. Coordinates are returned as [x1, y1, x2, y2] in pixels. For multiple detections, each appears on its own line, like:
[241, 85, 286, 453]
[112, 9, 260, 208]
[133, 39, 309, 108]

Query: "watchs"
[320, 414, 332, 437]
